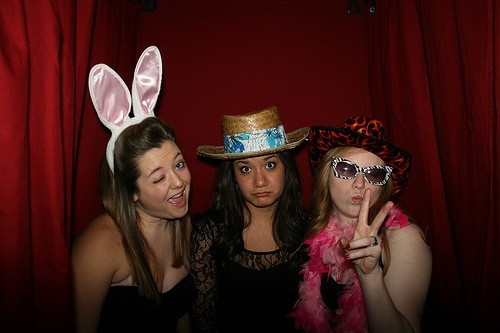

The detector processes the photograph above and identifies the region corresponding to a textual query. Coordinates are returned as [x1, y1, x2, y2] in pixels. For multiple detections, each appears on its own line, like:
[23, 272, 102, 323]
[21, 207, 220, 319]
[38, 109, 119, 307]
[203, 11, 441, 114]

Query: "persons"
[71, 116, 206, 333]
[287, 112, 433, 332]
[189, 104, 310, 333]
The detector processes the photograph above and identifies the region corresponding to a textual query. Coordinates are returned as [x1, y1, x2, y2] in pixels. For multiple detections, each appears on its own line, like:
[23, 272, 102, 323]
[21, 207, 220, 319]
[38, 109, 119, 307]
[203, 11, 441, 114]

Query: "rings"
[370, 236, 378, 247]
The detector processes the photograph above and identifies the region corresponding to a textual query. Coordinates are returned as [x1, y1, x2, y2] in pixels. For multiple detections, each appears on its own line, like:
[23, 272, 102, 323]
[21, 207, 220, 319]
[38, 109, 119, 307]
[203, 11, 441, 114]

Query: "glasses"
[328, 156, 394, 186]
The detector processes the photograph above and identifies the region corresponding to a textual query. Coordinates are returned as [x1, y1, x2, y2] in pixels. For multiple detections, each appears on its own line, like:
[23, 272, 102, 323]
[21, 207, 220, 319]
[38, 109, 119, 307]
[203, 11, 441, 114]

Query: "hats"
[196, 106, 310, 159]
[310, 116, 413, 203]
